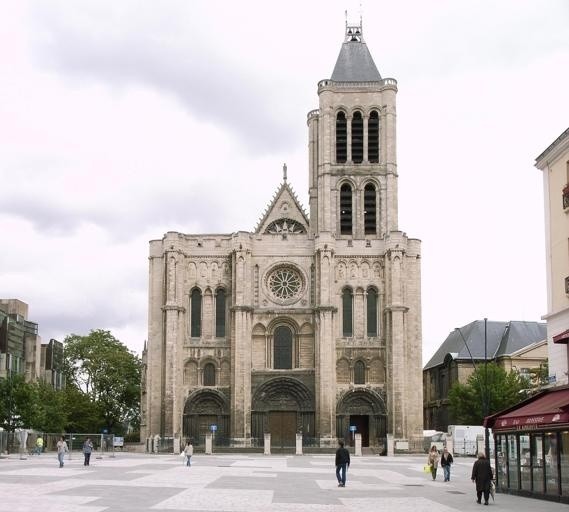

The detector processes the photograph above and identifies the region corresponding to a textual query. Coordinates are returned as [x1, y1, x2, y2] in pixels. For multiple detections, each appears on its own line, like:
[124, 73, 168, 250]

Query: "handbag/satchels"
[424, 465, 433, 472]
[180, 452, 186, 459]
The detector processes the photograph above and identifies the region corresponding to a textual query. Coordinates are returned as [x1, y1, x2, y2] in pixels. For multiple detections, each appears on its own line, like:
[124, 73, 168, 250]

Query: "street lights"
[455, 318, 509, 463]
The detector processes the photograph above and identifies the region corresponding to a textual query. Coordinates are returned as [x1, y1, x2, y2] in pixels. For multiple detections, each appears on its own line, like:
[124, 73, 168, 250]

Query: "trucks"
[430, 425, 529, 458]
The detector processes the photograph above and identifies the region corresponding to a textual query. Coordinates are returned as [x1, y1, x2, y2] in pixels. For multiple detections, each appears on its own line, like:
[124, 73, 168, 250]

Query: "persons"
[82, 437, 94, 467]
[441, 447, 454, 482]
[56, 436, 70, 469]
[334, 440, 351, 487]
[428, 446, 440, 481]
[26, 433, 44, 455]
[471, 452, 493, 505]
[184, 441, 193, 467]
